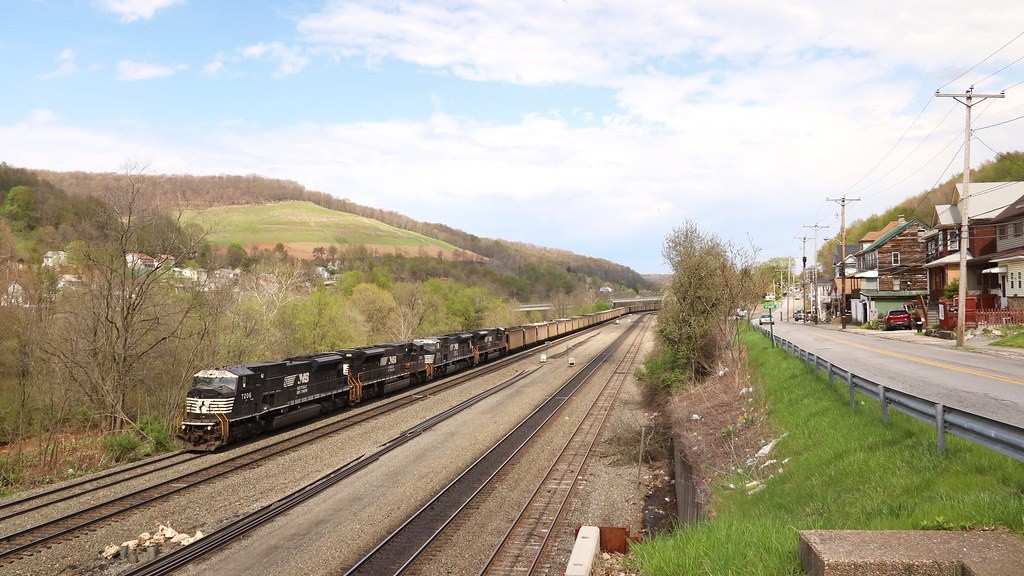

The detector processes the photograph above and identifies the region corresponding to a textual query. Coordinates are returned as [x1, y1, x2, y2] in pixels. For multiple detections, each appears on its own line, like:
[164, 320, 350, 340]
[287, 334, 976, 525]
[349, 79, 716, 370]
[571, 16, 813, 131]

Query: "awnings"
[835, 271, 879, 279]
[982, 265, 1007, 273]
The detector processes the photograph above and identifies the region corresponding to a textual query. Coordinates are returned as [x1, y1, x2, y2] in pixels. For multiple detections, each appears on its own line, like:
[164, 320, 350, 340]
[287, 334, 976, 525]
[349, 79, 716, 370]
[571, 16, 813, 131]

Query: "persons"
[914, 313, 922, 333]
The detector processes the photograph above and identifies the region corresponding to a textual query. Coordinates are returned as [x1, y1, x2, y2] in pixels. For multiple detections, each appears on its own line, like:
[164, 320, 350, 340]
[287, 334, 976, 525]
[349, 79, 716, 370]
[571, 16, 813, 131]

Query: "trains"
[175, 303, 663, 451]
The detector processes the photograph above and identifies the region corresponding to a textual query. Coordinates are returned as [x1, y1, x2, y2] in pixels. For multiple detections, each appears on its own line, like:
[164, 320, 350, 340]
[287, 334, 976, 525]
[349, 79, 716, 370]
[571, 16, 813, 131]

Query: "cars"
[793, 310, 805, 320]
[795, 295, 802, 300]
[759, 314, 774, 325]
[806, 310, 815, 321]
[883, 309, 913, 330]
[765, 293, 774, 301]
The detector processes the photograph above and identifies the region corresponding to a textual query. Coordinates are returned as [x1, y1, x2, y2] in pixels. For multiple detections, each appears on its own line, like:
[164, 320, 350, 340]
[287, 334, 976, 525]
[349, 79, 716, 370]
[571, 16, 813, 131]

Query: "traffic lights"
[803, 257, 806, 262]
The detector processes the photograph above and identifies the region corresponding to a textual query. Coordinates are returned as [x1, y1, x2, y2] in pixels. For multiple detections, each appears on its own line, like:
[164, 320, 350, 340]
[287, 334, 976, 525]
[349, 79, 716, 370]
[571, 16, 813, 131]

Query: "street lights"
[824, 237, 846, 329]
